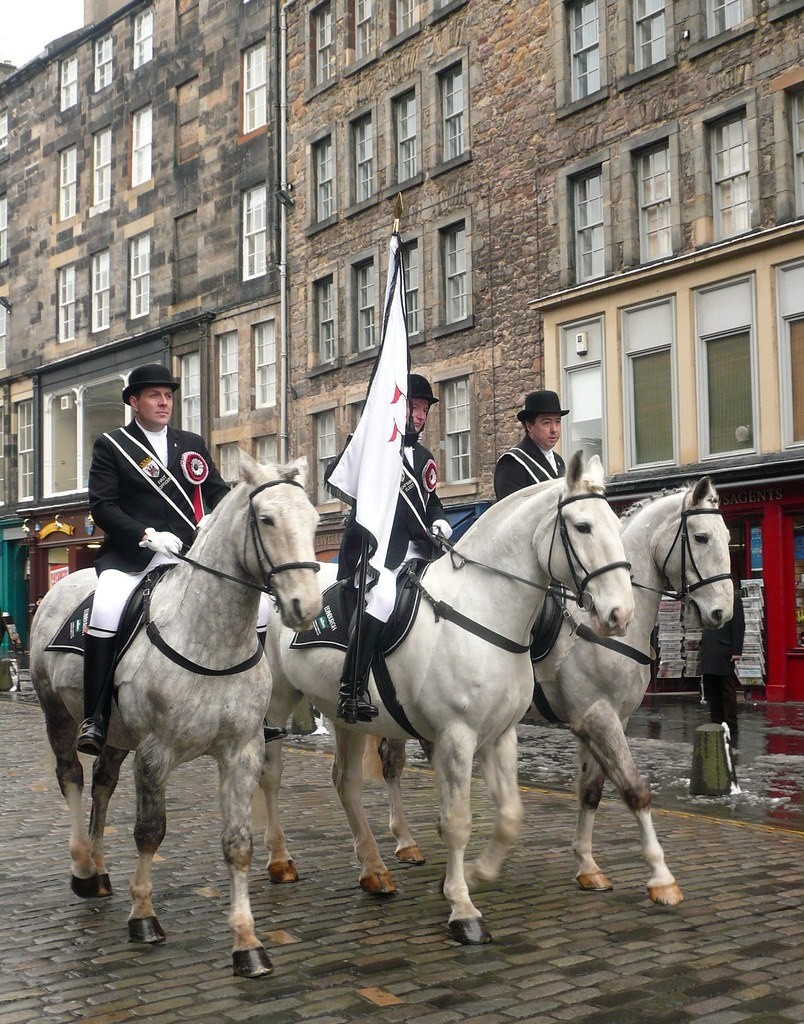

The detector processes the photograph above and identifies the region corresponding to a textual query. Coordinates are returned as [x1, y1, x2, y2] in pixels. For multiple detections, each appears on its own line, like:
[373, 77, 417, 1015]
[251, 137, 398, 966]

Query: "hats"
[122, 364, 180, 406]
[407, 374, 439, 405]
[517, 391, 570, 422]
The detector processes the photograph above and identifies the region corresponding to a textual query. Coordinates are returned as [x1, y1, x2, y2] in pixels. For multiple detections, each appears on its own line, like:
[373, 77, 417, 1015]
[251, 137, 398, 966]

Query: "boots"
[336, 611, 387, 718]
[77, 631, 121, 755]
[263, 727, 288, 743]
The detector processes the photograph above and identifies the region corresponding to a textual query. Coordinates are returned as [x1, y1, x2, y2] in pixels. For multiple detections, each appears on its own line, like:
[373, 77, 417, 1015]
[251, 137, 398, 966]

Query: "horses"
[30, 445, 322, 981]
[258, 449, 734, 949]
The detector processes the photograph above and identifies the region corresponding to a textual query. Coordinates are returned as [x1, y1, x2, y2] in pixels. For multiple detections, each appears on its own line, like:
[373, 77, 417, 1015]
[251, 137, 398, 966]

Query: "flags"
[321, 232, 412, 593]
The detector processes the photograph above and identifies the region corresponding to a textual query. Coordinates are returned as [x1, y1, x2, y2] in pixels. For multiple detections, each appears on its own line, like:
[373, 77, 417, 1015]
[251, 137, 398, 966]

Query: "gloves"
[431, 519, 453, 540]
[139, 528, 184, 559]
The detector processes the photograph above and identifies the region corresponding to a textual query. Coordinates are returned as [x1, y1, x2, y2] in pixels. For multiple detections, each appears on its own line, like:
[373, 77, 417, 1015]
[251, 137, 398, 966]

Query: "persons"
[74, 362, 287, 755]
[698, 595, 745, 756]
[495, 391, 569, 500]
[335, 372, 453, 714]
